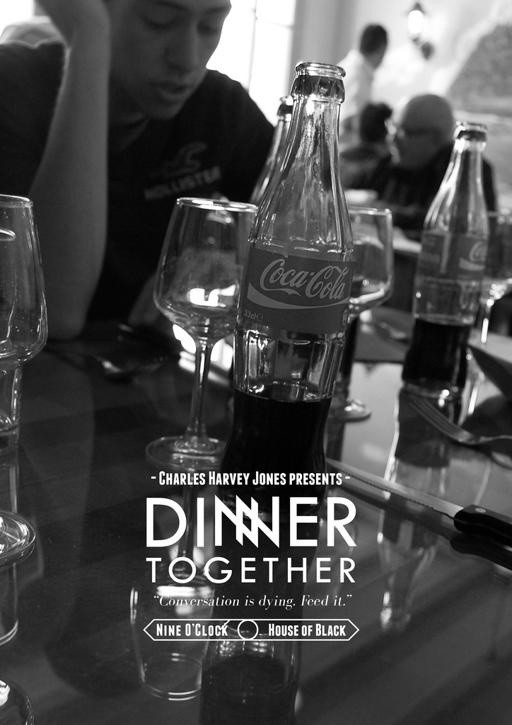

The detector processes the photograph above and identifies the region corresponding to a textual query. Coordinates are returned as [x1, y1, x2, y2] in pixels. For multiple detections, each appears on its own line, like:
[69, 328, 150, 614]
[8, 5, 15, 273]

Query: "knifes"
[322, 456, 512, 549]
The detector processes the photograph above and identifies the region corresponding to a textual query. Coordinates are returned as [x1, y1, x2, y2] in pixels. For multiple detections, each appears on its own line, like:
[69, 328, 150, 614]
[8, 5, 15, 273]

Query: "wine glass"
[143, 195, 260, 475]
[325, 204, 398, 424]
[127, 468, 219, 704]
[0, 191, 51, 562]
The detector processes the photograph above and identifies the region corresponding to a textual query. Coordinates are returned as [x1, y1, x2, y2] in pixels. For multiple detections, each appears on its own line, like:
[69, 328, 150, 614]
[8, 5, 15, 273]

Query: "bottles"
[375, 384, 465, 636]
[211, 60, 358, 527]
[401, 120, 492, 403]
[197, 526, 322, 724]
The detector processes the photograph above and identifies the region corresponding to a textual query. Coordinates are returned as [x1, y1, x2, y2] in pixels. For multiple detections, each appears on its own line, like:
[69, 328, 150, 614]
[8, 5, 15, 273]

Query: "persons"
[368, 93, 499, 232]
[0, 0, 278, 341]
[333, 24, 390, 117]
[339, 101, 395, 189]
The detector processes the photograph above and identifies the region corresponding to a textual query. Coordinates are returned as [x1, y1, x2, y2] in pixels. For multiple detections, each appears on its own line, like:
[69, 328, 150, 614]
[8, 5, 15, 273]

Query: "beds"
[0, 317, 512, 724]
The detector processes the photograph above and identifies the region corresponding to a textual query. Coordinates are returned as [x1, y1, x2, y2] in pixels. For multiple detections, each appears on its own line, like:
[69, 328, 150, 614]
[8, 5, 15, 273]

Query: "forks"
[407, 398, 512, 447]
